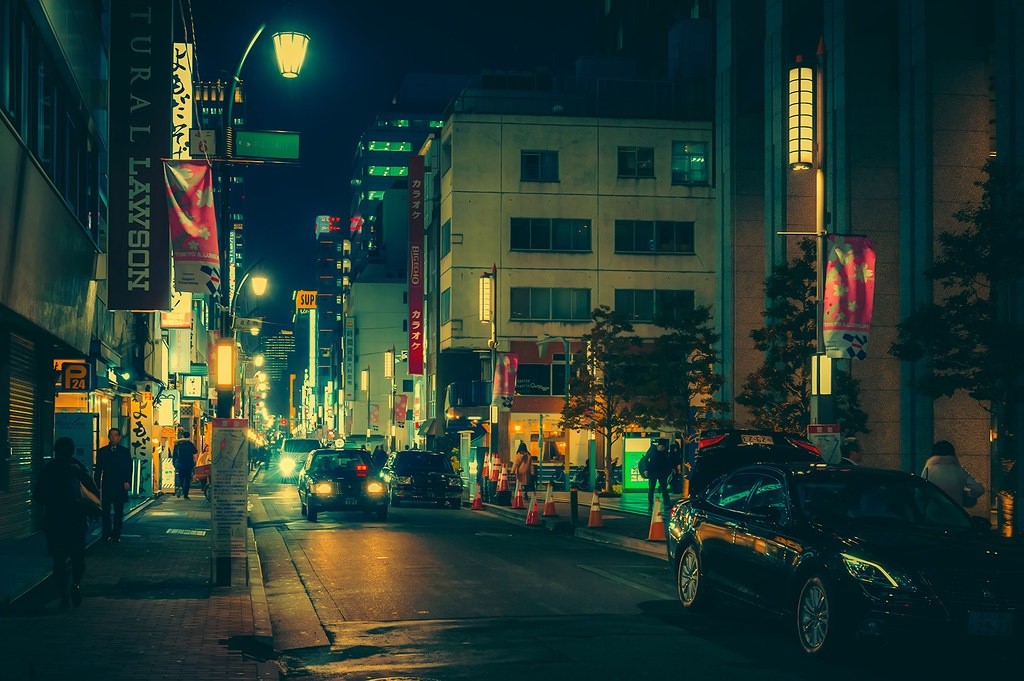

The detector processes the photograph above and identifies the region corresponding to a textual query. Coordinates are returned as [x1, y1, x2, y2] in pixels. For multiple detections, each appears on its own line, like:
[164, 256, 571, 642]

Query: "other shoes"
[184, 497, 190, 500]
[177, 487, 182, 498]
[102, 536, 110, 541]
[524, 494, 528, 500]
[62, 584, 82, 607]
[113, 536, 121, 543]
[648, 504, 653, 511]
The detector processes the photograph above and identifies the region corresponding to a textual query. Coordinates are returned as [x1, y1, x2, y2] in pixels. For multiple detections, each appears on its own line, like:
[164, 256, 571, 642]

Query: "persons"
[510, 443, 535, 500]
[34, 436, 101, 606]
[643, 438, 673, 511]
[360, 443, 418, 467]
[94, 428, 133, 545]
[921, 440, 985, 509]
[837, 437, 866, 467]
[172, 432, 198, 500]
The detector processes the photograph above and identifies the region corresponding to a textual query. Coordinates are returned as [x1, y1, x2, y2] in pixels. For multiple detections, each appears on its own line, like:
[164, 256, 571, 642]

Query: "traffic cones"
[470, 482, 484, 510]
[645, 497, 668, 542]
[482, 452, 501, 482]
[496, 464, 508, 491]
[524, 491, 538, 525]
[588, 491, 602, 528]
[510, 478, 528, 510]
[542, 484, 558, 516]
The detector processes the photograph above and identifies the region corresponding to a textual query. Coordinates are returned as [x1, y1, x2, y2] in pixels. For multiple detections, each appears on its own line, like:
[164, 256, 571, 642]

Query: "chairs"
[318, 460, 336, 472]
[347, 460, 361, 471]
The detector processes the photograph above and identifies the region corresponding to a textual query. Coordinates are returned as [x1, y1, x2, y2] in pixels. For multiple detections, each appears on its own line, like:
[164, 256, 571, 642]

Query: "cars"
[297, 450, 387, 523]
[380, 450, 465, 509]
[277, 438, 325, 483]
[665, 430, 1024, 659]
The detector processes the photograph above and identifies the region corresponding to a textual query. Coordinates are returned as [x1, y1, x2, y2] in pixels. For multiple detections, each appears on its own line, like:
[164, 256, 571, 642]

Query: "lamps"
[116, 371, 130, 380]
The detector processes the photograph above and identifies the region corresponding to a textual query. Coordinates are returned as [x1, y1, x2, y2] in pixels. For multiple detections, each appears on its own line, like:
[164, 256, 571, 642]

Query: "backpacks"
[639, 454, 650, 478]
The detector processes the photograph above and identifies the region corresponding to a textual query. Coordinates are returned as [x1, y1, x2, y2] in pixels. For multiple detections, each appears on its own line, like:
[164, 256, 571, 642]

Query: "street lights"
[217, 2, 313, 583]
